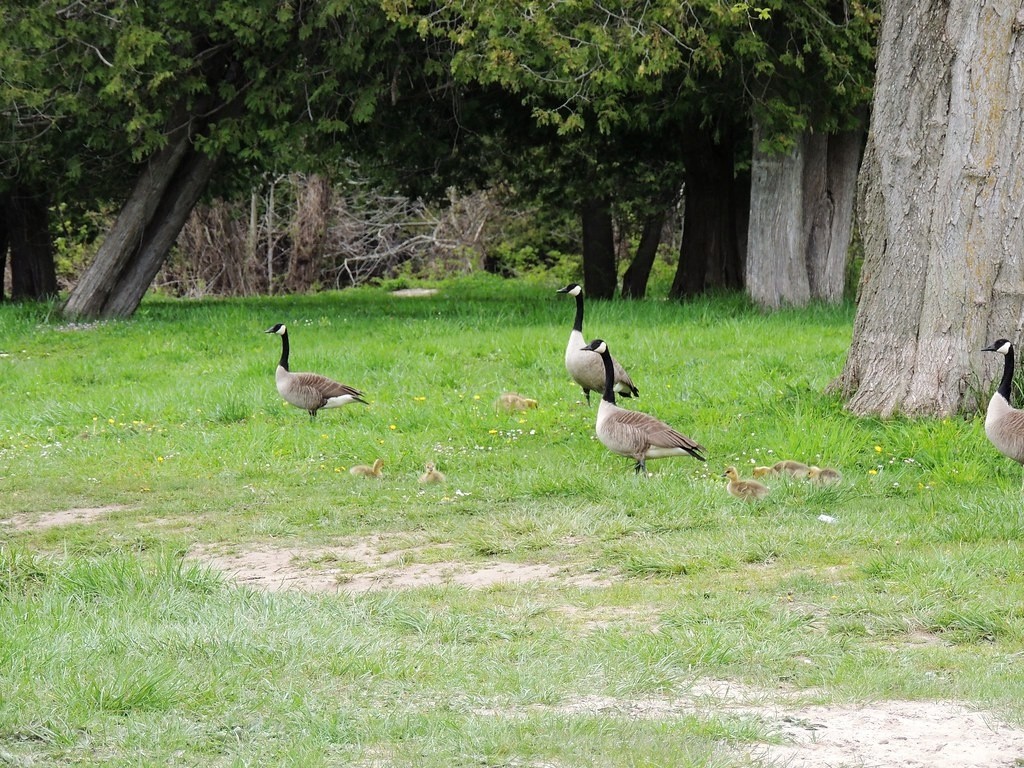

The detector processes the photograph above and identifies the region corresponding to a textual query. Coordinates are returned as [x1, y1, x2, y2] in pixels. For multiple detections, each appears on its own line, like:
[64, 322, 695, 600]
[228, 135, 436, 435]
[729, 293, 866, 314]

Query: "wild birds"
[721, 466, 770, 499]
[752, 460, 842, 485]
[264, 323, 366, 423]
[422, 462, 446, 483]
[350, 457, 385, 476]
[981, 339, 1023, 465]
[556, 283, 640, 407]
[493, 392, 538, 410]
[579, 340, 708, 475]
